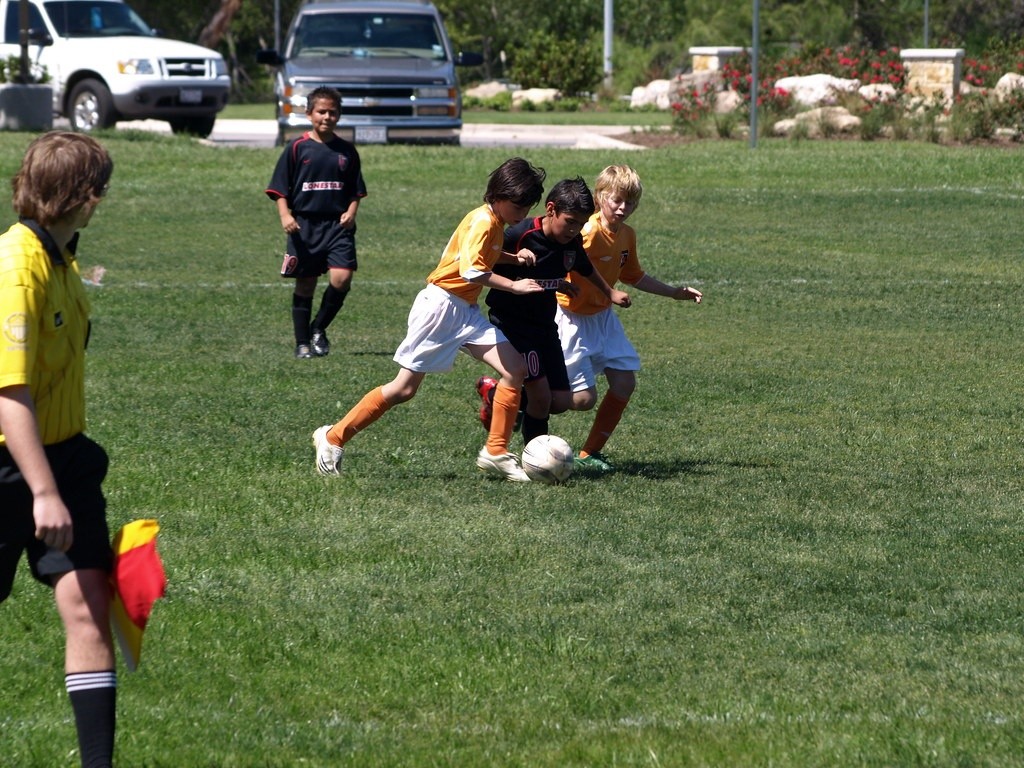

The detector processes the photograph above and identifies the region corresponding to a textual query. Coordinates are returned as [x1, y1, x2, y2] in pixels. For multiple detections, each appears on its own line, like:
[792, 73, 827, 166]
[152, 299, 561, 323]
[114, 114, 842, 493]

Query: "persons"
[0, 133, 120, 768]
[476, 175, 632, 452]
[555, 164, 702, 473]
[313, 155, 545, 483]
[264, 85, 367, 358]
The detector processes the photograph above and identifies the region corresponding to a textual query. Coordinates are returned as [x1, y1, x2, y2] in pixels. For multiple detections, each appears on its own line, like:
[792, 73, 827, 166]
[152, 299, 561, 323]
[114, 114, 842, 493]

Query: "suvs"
[255, 0, 484, 146]
[1, 0, 232, 139]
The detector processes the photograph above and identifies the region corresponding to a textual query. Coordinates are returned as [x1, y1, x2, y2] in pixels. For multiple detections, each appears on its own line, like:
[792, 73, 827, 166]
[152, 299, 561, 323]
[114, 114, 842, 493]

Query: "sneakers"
[477, 376, 498, 432]
[573, 451, 616, 475]
[477, 445, 531, 482]
[295, 344, 313, 358]
[310, 328, 329, 356]
[512, 385, 525, 432]
[312, 425, 345, 477]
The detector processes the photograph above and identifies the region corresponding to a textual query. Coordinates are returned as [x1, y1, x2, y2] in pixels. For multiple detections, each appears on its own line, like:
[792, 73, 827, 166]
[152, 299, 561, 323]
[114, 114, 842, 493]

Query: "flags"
[104, 518, 165, 673]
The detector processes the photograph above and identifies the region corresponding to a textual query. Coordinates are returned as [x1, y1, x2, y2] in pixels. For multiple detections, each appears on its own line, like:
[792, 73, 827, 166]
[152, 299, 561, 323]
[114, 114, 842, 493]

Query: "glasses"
[101, 182, 110, 196]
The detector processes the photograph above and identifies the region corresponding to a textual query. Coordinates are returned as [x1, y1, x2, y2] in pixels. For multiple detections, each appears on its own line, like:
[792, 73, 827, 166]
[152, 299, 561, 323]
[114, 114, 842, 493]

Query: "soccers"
[517, 432, 578, 483]
